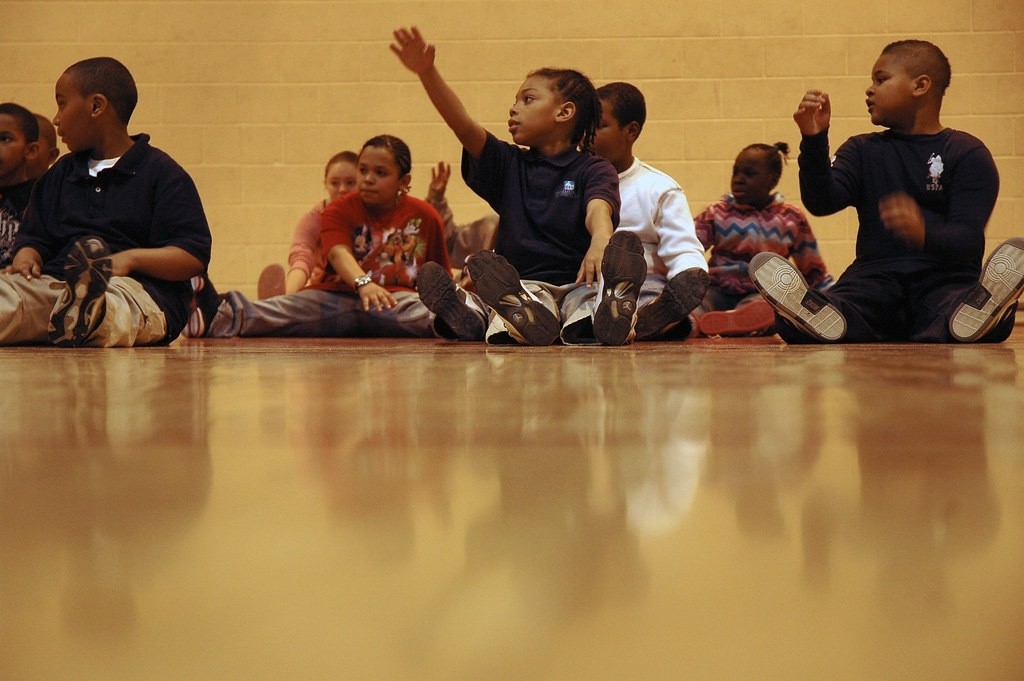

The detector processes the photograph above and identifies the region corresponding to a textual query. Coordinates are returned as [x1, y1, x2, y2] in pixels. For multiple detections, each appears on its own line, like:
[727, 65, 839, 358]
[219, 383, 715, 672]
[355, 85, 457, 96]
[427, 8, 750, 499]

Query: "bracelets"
[354, 275, 371, 288]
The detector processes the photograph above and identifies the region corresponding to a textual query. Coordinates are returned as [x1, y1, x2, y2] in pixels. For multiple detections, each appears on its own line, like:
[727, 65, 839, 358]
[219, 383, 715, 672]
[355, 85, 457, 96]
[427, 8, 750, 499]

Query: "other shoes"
[47, 235, 113, 348]
[634, 267, 710, 340]
[181, 272, 223, 338]
[700, 300, 775, 335]
[948, 237, 1024, 342]
[747, 251, 848, 343]
[257, 264, 286, 300]
[464, 249, 560, 346]
[416, 261, 489, 342]
[593, 230, 647, 346]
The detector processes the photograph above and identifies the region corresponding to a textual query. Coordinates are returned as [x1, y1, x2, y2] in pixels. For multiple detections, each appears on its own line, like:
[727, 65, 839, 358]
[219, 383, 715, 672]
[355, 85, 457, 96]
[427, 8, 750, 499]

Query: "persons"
[0, 57, 212, 347]
[186, 134, 490, 343]
[749, 39, 1024, 343]
[581, 82, 710, 339]
[388, 26, 646, 345]
[694, 142, 836, 337]
